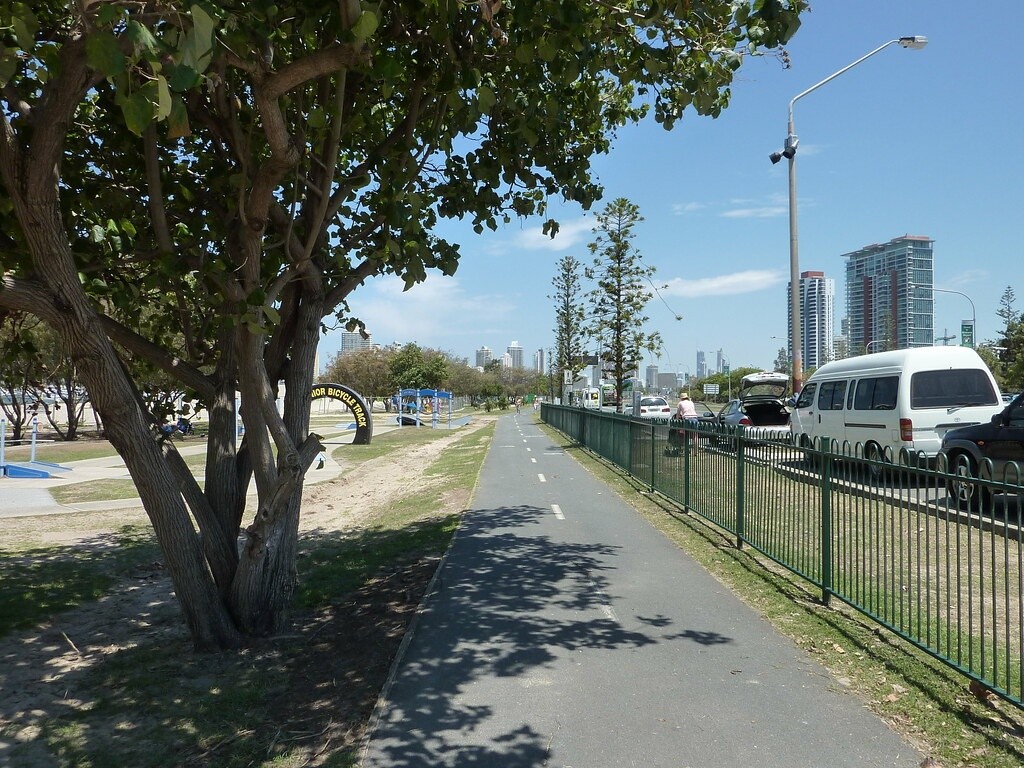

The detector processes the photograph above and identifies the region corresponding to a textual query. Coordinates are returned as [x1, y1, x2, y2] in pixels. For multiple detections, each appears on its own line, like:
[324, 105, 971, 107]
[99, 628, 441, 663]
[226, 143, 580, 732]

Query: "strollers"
[664, 412, 692, 457]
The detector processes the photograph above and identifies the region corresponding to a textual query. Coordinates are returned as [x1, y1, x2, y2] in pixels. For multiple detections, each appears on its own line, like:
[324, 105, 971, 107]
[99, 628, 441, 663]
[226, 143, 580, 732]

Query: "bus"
[582, 388, 600, 410]
[601, 384, 618, 406]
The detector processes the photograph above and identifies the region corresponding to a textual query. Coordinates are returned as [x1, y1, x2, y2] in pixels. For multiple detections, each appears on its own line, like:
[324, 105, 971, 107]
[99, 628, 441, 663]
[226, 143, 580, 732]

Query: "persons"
[676, 393, 698, 456]
[515, 399, 521, 414]
[533, 395, 538, 410]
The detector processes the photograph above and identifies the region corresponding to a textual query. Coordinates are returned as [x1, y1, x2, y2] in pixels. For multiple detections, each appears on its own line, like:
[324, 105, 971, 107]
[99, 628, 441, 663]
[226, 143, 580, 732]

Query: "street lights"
[866, 338, 890, 355]
[768, 33, 928, 396]
[709, 351, 730, 402]
[910, 285, 976, 350]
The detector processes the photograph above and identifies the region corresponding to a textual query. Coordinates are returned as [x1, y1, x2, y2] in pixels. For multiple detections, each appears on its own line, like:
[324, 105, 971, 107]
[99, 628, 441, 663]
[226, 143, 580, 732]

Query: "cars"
[936, 389, 1024, 513]
[1000, 394, 1020, 408]
[694, 371, 791, 448]
[623, 397, 670, 426]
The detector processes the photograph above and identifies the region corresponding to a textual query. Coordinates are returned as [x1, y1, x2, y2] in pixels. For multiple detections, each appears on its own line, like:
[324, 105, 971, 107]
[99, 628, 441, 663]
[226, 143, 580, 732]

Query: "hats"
[680, 393, 688, 398]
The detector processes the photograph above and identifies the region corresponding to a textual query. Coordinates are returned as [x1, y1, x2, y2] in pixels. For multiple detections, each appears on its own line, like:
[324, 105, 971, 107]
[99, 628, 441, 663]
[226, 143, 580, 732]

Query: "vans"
[788, 345, 1004, 485]
